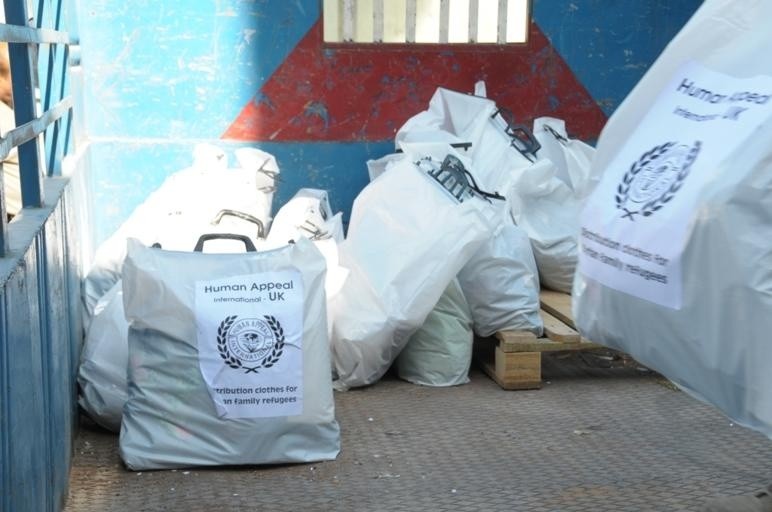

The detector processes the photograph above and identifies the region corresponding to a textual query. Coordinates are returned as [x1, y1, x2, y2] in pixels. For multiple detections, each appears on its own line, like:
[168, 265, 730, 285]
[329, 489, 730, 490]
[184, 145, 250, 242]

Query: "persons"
[0, 41, 23, 223]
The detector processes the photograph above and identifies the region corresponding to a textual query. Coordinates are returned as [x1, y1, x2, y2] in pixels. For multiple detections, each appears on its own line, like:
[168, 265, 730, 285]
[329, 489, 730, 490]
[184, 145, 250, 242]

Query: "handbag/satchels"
[509, 115, 595, 292]
[334, 152, 497, 387]
[395, 88, 536, 174]
[459, 226, 543, 337]
[118, 233, 341, 472]
[572, 0, 771, 436]
[76, 209, 265, 432]
[80, 139, 281, 322]
[395, 289, 472, 388]
